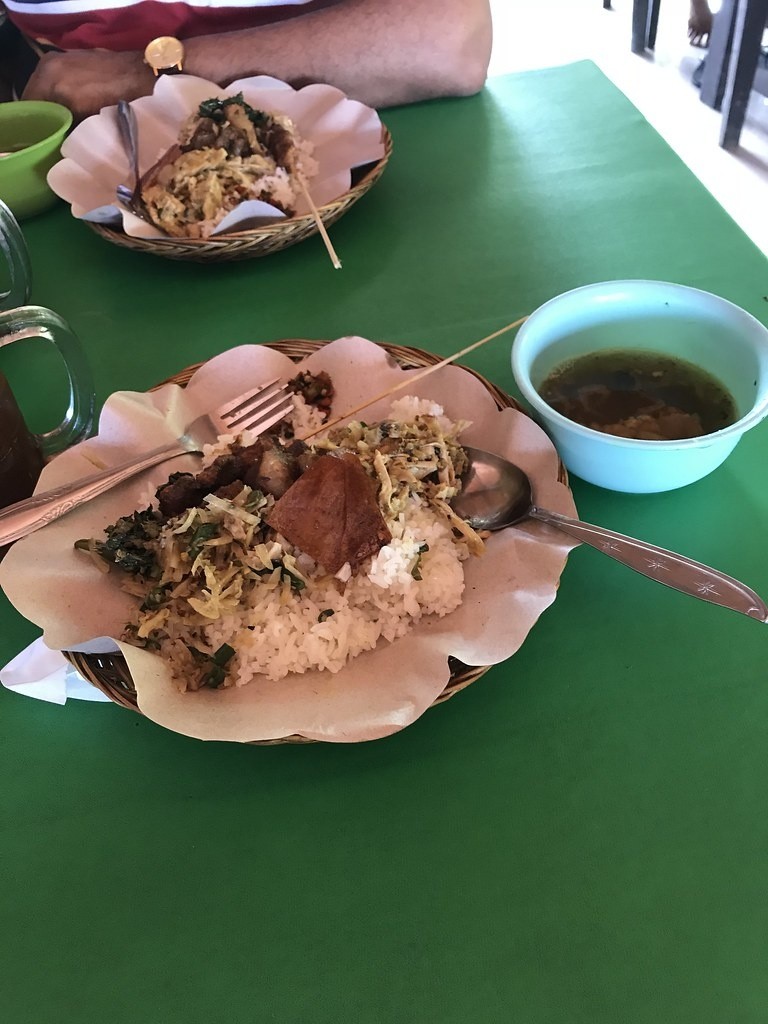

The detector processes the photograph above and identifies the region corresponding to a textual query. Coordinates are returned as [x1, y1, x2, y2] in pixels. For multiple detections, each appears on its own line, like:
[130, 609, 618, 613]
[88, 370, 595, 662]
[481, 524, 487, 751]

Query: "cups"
[0, 198, 34, 311]
[0, 306, 96, 561]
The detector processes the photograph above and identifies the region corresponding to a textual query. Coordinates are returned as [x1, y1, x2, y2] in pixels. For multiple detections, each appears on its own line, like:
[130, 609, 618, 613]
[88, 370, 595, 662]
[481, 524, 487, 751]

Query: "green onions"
[188, 642, 237, 688]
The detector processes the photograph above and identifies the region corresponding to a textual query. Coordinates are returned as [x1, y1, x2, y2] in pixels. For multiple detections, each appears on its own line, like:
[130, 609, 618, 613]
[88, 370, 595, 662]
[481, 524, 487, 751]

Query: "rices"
[193, 113, 320, 238]
[151, 394, 464, 685]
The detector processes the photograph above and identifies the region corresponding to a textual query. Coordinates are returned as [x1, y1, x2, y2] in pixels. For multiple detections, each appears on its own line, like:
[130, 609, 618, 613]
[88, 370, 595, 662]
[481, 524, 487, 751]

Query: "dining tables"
[0, 56, 768, 1024]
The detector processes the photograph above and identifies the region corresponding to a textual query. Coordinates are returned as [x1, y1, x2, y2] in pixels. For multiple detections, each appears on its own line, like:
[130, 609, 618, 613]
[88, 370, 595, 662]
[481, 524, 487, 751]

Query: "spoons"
[113, 112, 136, 212]
[421, 444, 768, 626]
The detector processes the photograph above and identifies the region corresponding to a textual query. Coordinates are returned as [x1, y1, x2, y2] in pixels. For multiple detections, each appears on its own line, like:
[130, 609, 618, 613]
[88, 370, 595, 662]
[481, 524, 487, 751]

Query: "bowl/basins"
[510, 279, 768, 494]
[0, 99, 74, 220]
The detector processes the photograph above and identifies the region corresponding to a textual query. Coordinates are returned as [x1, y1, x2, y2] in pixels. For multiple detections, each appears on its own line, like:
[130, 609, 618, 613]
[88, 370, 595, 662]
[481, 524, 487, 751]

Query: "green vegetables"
[198, 91, 268, 127]
[73, 490, 264, 613]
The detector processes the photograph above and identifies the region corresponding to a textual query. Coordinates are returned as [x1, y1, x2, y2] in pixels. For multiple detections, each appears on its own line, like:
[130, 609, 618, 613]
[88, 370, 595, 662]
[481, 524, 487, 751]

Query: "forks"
[0, 375, 295, 547]
[118, 100, 156, 225]
